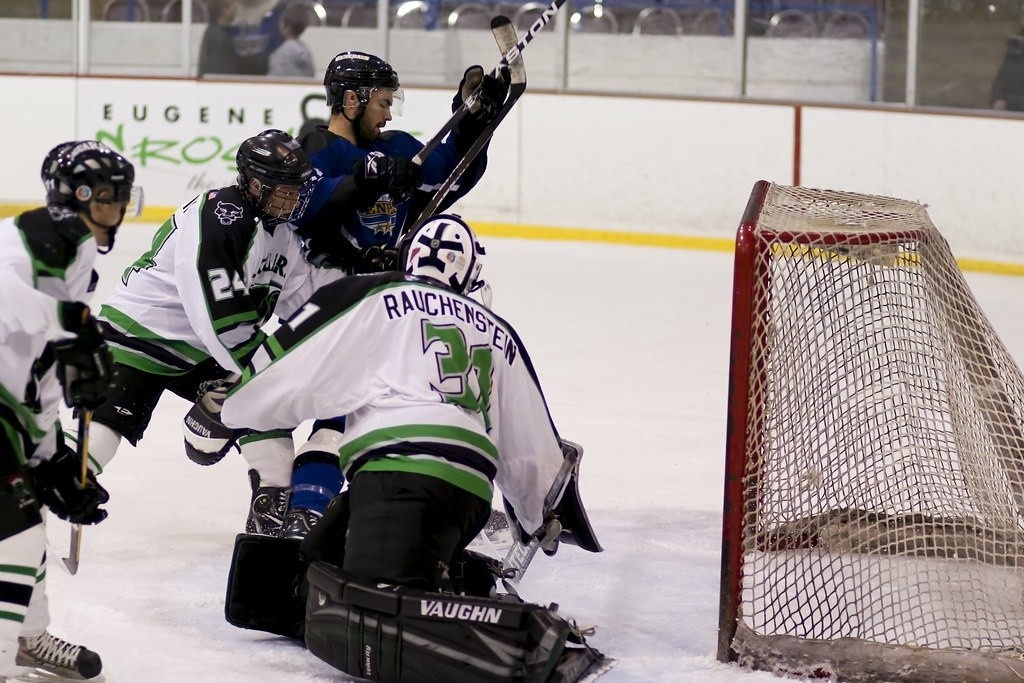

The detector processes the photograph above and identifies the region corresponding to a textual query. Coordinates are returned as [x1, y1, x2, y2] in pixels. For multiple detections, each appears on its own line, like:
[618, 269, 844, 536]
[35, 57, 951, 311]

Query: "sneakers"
[15, 631, 102, 679]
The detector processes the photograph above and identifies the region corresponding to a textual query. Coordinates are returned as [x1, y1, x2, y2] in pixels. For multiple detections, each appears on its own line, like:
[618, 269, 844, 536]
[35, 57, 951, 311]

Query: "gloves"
[55, 300, 119, 419]
[23, 420, 109, 525]
[448, 65, 512, 172]
[354, 151, 425, 206]
[183, 390, 249, 465]
[520, 517, 561, 556]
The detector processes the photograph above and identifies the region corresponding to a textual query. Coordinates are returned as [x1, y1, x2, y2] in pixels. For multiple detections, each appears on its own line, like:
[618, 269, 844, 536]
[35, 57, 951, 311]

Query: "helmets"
[237, 129, 324, 193]
[40, 139, 134, 214]
[323, 51, 401, 105]
[403, 213, 487, 296]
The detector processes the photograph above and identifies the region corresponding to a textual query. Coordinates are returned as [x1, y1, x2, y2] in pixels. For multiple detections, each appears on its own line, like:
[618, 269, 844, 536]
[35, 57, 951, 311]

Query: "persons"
[65, 44, 513, 478]
[1, 142, 138, 670]
[197, 0, 317, 77]
[180, 213, 611, 683]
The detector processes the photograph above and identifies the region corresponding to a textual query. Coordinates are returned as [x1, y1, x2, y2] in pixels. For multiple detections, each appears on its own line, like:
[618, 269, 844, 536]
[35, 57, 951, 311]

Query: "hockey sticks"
[408, 0, 568, 166]
[481, 451, 578, 584]
[44, 406, 92, 577]
[396, 15, 529, 253]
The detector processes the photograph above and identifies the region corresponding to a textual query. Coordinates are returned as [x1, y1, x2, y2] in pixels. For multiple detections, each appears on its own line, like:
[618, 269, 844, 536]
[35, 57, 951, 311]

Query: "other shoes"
[545, 617, 617, 683]
[276, 506, 323, 541]
[246, 469, 292, 540]
